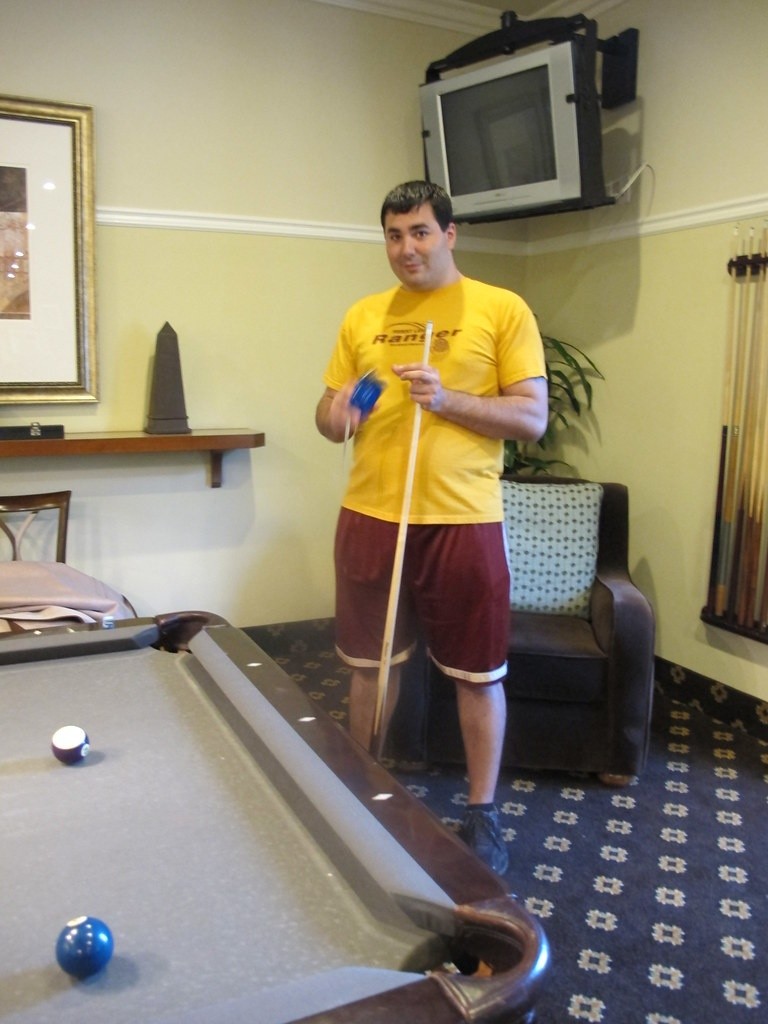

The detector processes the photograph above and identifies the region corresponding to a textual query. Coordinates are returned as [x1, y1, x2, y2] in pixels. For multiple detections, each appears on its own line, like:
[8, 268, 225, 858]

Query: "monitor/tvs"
[419, 40, 607, 224]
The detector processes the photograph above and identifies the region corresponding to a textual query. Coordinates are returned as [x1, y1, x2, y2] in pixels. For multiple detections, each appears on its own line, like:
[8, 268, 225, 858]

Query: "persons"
[314, 180, 549, 877]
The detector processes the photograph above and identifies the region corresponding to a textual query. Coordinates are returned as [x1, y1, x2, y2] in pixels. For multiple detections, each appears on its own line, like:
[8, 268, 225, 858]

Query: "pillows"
[501, 479, 604, 622]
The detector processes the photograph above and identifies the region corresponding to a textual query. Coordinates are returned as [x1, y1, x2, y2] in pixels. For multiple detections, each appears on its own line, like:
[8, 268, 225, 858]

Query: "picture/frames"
[0, 95, 101, 406]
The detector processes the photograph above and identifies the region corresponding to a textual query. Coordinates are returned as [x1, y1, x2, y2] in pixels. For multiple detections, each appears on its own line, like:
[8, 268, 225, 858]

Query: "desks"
[0, 611, 550, 1024]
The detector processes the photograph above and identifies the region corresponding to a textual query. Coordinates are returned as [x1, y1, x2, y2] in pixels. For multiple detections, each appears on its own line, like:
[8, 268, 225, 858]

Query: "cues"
[368, 322, 434, 759]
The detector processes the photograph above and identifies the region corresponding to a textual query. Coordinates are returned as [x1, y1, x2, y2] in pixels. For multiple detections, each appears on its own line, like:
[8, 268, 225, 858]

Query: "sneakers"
[460, 805, 510, 877]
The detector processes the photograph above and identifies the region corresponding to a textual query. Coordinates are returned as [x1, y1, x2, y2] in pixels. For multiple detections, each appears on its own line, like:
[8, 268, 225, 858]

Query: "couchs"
[398, 475, 656, 788]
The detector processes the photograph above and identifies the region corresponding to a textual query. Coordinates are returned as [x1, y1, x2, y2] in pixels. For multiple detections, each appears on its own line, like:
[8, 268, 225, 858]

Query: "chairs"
[0, 490, 73, 563]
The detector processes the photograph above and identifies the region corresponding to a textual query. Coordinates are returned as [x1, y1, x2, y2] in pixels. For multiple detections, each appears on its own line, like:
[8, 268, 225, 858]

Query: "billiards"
[55, 915, 115, 980]
[51, 725, 91, 764]
